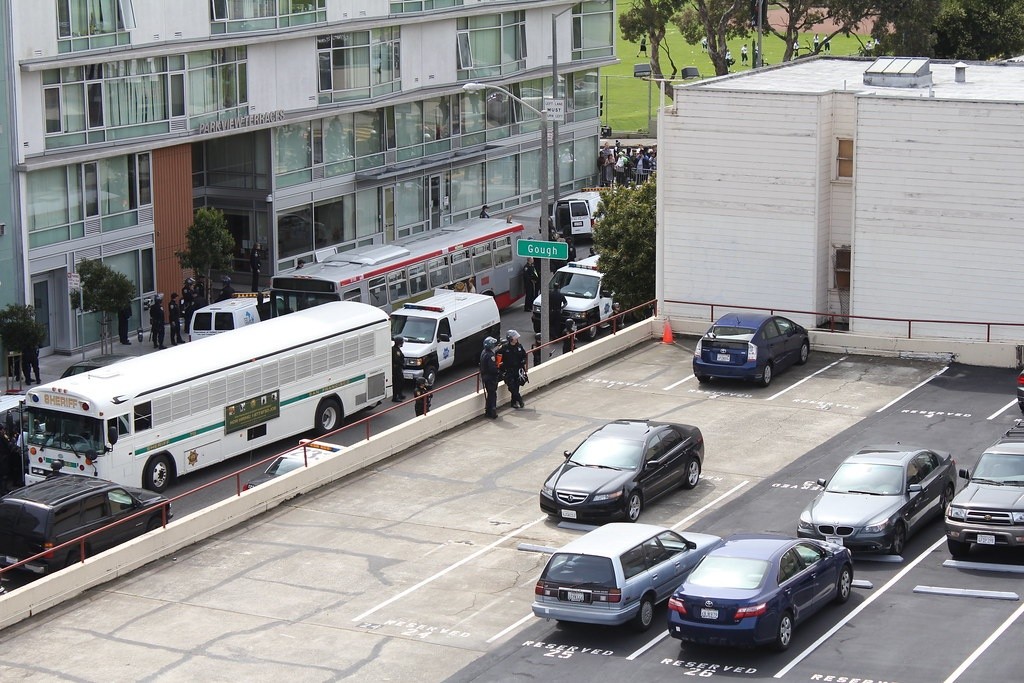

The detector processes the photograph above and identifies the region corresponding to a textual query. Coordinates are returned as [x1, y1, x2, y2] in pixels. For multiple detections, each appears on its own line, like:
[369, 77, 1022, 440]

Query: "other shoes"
[519, 399, 524, 408]
[171, 340, 176, 345]
[511, 403, 519, 408]
[491, 408, 496, 419]
[177, 337, 185, 343]
[486, 414, 498, 418]
[392, 395, 406, 402]
[154, 343, 158, 348]
[120, 340, 132, 345]
[16, 376, 40, 385]
[159, 345, 167, 349]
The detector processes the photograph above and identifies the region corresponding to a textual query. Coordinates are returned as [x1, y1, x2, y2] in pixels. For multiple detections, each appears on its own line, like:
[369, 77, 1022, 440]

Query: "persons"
[634, 30, 882, 68]
[531, 333, 543, 366]
[561, 318, 578, 354]
[497, 329, 527, 409]
[14, 332, 42, 385]
[117, 86, 491, 351]
[479, 335, 503, 419]
[557, 142, 658, 187]
[414, 376, 433, 417]
[0, 412, 53, 494]
[548, 282, 568, 341]
[608, 302, 625, 334]
[539, 213, 577, 276]
[392, 336, 407, 403]
[523, 256, 539, 312]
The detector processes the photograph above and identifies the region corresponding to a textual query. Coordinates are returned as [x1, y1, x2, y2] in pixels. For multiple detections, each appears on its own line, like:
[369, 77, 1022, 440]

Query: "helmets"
[612, 302, 620, 310]
[394, 337, 408, 346]
[535, 333, 542, 341]
[416, 377, 427, 385]
[565, 319, 575, 328]
[154, 274, 232, 303]
[483, 336, 497, 348]
[505, 329, 520, 340]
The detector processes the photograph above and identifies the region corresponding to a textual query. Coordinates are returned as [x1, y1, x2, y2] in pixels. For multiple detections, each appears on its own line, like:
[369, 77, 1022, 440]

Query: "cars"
[691, 311, 809, 388]
[666, 529, 854, 653]
[940, 441, 1024, 563]
[241, 439, 347, 493]
[530, 523, 724, 633]
[539, 418, 704, 523]
[797, 444, 958, 556]
[57, 354, 139, 381]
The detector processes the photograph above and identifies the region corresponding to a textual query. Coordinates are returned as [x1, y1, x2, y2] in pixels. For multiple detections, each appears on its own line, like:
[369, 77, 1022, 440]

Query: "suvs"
[0, 473, 173, 584]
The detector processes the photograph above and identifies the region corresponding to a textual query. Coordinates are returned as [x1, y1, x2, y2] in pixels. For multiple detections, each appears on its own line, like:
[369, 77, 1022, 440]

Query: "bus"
[267, 216, 536, 348]
[30, 299, 395, 492]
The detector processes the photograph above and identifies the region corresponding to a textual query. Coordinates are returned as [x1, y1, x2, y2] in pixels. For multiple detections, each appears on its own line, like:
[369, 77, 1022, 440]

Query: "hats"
[298, 260, 306, 264]
[483, 205, 490, 209]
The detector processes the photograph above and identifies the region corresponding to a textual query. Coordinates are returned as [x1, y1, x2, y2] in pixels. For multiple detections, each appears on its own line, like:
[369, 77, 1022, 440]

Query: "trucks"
[390, 290, 503, 389]
[548, 191, 604, 243]
[188, 296, 286, 343]
[532, 251, 624, 342]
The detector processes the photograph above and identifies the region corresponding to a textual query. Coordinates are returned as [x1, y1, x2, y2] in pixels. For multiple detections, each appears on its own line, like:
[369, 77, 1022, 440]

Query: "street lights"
[6, 400, 30, 487]
[463, 81, 553, 367]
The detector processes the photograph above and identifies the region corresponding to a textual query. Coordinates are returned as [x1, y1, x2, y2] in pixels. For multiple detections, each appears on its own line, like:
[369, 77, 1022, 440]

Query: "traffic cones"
[659, 321, 677, 345]
